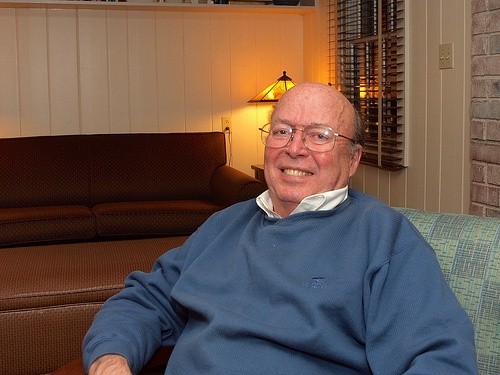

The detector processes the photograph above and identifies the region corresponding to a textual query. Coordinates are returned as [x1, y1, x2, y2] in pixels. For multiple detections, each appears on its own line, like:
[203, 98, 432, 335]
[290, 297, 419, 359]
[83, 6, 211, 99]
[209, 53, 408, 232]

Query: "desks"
[251, 164, 265, 187]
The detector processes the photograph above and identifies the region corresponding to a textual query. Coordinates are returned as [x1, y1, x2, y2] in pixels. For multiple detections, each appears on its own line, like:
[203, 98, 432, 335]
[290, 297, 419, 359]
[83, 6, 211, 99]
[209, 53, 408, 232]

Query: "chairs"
[49, 206, 500, 375]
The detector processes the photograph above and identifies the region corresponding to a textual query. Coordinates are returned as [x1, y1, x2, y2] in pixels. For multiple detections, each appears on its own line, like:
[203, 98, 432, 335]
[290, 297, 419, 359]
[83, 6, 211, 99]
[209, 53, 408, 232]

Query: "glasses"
[259, 122, 355, 152]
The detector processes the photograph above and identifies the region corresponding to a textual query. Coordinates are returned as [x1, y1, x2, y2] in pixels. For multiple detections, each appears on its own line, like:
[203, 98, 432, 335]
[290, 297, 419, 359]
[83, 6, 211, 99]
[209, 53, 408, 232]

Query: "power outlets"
[223, 117, 231, 133]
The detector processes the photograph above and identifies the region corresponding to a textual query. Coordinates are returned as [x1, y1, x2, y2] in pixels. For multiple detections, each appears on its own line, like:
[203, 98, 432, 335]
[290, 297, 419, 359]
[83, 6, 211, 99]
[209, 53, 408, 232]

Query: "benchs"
[0, 235, 188, 375]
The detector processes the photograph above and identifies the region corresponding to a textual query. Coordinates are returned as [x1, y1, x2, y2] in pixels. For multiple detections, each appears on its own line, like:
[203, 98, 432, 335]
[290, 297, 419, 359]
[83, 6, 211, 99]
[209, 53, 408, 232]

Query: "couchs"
[0, 132, 266, 245]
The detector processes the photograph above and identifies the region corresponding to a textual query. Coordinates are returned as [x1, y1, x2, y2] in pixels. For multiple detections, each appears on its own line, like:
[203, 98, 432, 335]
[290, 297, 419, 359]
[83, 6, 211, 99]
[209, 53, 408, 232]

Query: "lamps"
[247, 71, 298, 108]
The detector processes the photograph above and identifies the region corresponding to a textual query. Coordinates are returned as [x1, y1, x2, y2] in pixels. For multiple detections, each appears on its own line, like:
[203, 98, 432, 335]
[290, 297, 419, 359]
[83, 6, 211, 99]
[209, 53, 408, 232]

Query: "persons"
[81, 82, 477, 375]
